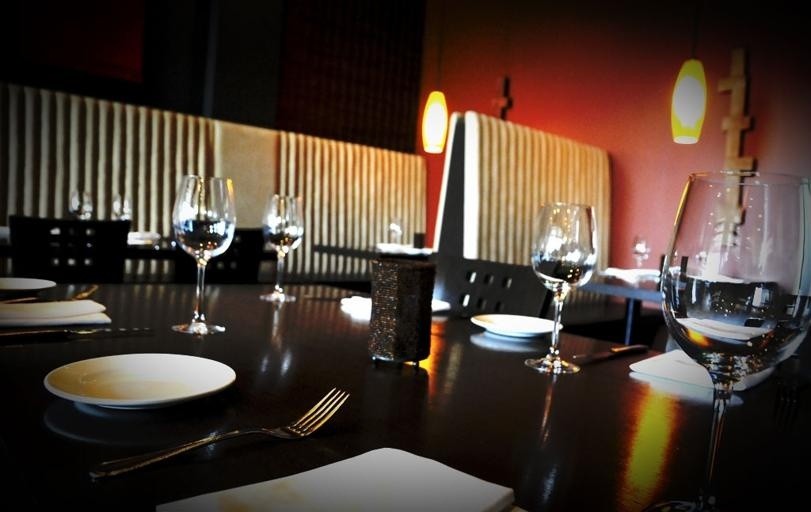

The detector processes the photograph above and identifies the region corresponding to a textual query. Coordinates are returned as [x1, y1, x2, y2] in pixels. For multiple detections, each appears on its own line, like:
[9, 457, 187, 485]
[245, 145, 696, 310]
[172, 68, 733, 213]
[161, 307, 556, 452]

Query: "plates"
[469, 334, 553, 354]
[0, 277, 57, 290]
[470, 313, 564, 339]
[43, 352, 236, 411]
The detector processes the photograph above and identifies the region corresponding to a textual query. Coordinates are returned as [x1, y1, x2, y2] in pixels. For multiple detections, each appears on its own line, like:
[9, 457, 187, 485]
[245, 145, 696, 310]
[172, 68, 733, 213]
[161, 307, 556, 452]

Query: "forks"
[88, 386, 353, 478]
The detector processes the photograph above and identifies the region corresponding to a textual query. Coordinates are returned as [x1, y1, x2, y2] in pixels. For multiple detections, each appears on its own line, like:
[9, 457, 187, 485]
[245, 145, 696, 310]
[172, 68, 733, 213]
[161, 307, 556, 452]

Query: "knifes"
[573, 343, 648, 365]
[0, 326, 157, 338]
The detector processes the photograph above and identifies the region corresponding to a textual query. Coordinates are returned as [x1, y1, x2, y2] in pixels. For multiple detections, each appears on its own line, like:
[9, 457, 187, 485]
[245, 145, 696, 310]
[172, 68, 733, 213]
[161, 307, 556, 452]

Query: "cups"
[65, 188, 132, 223]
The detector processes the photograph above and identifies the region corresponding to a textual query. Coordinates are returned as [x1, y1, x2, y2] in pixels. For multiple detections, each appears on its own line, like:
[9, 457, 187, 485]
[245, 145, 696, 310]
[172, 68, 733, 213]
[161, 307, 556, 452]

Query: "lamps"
[421, 1, 449, 155]
[669, 0, 708, 145]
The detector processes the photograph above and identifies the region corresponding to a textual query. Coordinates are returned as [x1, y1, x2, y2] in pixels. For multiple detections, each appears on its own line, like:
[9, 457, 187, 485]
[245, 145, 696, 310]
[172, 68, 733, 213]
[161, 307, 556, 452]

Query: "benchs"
[458, 106, 674, 350]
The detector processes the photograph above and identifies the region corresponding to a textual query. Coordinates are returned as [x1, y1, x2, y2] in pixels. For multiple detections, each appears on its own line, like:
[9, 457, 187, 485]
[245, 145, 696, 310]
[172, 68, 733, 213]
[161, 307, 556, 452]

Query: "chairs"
[8, 214, 264, 284]
[441, 259, 560, 322]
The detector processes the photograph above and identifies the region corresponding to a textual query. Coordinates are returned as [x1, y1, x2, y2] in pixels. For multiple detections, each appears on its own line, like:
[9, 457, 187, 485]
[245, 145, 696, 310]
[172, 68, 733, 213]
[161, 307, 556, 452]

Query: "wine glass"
[633, 236, 651, 270]
[645, 169, 811, 512]
[258, 193, 305, 302]
[523, 201, 600, 375]
[170, 173, 237, 334]
